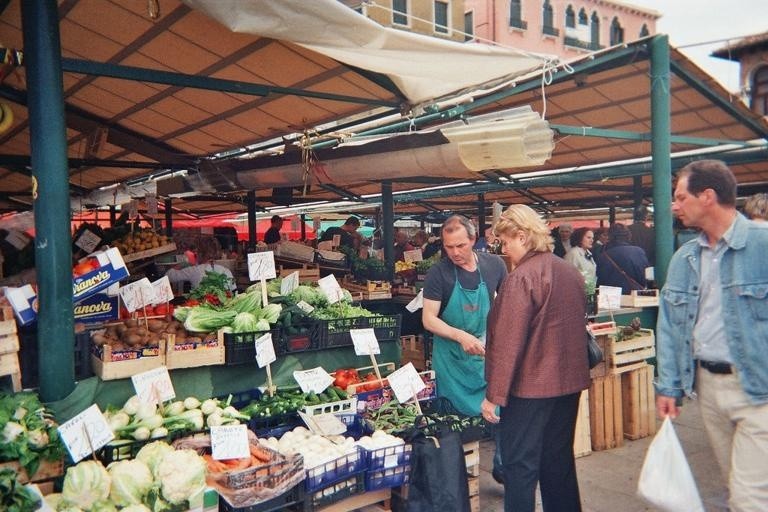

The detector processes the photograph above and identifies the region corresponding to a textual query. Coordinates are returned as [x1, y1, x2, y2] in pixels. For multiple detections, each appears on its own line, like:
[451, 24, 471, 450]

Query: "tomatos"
[332, 368, 390, 394]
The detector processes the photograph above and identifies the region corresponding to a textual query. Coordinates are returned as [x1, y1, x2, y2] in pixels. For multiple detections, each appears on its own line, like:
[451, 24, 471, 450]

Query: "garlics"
[110, 394, 238, 440]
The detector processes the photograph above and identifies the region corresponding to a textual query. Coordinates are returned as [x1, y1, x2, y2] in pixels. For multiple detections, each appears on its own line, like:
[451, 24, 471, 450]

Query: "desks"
[586, 302, 659, 334]
[1, 334, 403, 429]
[317, 483, 391, 510]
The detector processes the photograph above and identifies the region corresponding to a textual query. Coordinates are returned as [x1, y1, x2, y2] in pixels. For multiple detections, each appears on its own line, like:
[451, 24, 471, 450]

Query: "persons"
[650, 157, 768, 512]
[598, 222, 648, 298]
[318, 217, 441, 280]
[564, 226, 596, 302]
[628, 205, 653, 266]
[553, 224, 573, 257]
[164, 236, 238, 298]
[263, 215, 283, 244]
[422, 215, 508, 487]
[739, 190, 768, 222]
[591, 227, 609, 264]
[481, 203, 588, 511]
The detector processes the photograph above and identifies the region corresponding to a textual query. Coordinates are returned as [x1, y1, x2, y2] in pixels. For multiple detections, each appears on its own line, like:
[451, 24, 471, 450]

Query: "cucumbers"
[267, 295, 308, 334]
[237, 386, 349, 417]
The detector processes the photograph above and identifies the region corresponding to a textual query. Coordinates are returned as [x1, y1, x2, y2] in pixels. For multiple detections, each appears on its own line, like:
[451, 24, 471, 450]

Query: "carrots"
[203, 443, 292, 481]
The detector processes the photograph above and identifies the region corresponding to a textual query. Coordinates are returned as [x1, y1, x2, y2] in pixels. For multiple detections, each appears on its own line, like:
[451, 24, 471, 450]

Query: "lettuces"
[172, 291, 282, 343]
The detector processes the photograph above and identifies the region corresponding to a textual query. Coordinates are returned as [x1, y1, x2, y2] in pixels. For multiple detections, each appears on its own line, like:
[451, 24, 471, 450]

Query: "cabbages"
[43, 440, 207, 512]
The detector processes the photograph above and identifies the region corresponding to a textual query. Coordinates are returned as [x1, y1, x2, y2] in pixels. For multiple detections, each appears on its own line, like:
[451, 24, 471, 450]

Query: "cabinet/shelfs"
[2, 228, 429, 316]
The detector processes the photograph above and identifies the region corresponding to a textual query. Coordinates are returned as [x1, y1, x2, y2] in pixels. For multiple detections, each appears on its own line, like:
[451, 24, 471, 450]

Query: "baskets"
[94, 363, 488, 512]
[314, 250, 431, 285]
[224, 313, 403, 360]
[94, 366, 488, 512]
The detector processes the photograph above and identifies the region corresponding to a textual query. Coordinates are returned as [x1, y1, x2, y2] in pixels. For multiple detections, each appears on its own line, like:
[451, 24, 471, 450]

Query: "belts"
[694, 358, 735, 376]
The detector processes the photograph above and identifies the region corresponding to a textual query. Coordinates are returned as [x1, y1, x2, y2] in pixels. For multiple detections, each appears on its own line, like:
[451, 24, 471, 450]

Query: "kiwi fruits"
[93, 319, 216, 352]
